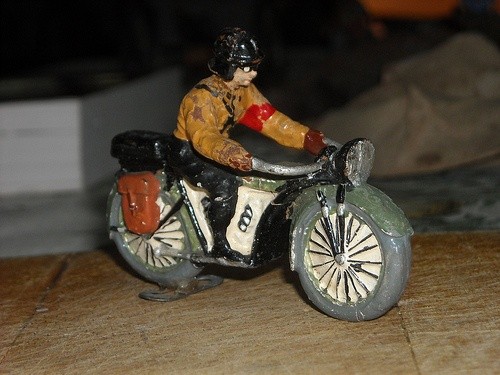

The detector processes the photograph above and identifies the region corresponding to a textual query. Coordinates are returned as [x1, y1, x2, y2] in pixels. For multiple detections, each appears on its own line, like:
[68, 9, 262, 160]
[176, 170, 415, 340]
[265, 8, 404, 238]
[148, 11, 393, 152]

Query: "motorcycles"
[105, 127, 414, 322]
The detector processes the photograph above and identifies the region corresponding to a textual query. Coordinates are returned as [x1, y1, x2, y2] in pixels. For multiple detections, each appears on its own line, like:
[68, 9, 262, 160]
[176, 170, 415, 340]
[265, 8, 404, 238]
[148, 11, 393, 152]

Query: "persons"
[172, 27, 326, 261]
